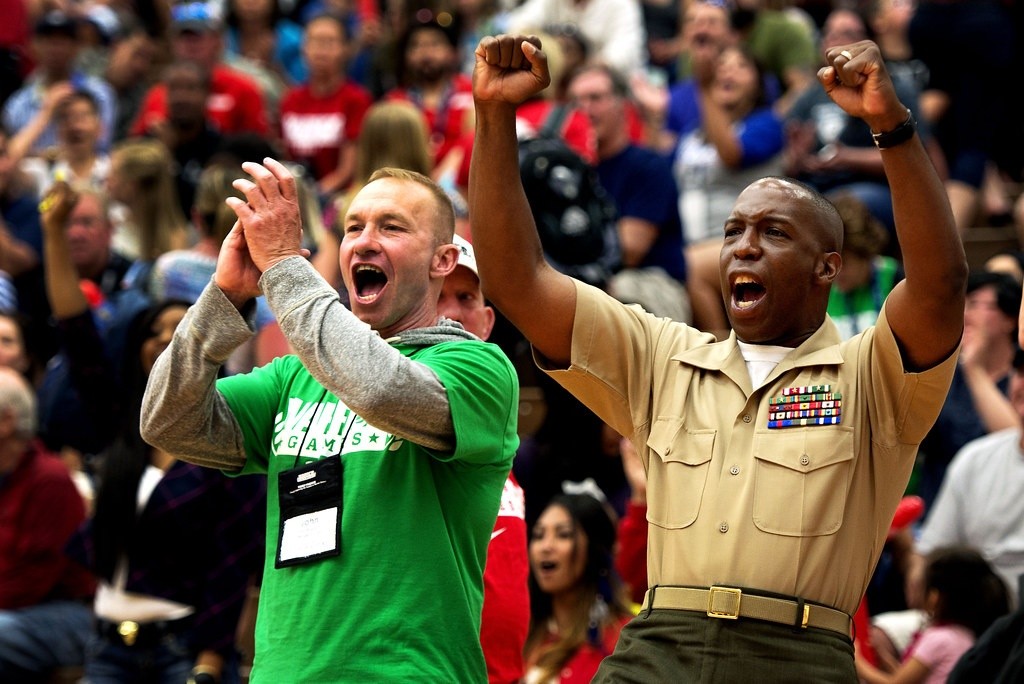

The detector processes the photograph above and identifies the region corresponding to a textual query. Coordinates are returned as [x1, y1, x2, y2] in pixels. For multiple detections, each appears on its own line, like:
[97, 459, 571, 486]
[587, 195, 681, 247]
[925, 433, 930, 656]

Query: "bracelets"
[870, 109, 917, 150]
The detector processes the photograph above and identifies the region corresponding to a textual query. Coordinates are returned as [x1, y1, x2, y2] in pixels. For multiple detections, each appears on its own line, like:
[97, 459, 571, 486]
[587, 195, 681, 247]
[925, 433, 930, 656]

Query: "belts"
[640, 582, 852, 640]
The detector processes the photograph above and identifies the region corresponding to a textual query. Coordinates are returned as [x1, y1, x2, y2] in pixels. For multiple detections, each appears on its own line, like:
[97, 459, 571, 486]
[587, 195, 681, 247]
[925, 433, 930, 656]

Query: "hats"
[81, 4, 121, 38]
[33, 10, 76, 39]
[520, 110, 602, 160]
[521, 147, 615, 280]
[172, 2, 213, 38]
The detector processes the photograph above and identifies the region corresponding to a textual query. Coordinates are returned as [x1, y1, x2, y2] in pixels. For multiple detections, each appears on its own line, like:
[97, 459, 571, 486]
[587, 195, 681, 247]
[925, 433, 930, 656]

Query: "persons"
[0, 0, 1024, 684]
[139, 156, 519, 684]
[469, 33, 970, 684]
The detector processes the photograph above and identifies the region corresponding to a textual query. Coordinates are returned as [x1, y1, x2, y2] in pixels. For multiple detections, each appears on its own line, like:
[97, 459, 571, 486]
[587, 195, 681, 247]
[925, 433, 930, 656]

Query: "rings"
[841, 51, 853, 60]
